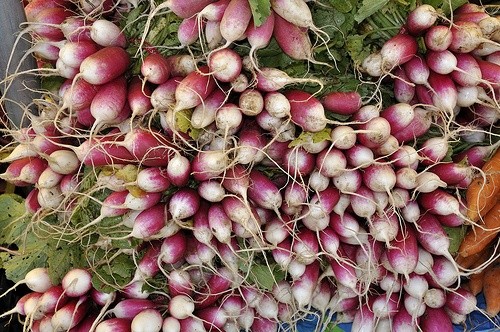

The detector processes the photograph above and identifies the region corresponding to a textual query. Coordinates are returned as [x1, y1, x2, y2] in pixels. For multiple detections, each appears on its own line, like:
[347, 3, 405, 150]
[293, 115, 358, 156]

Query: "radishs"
[0, 0, 499, 331]
